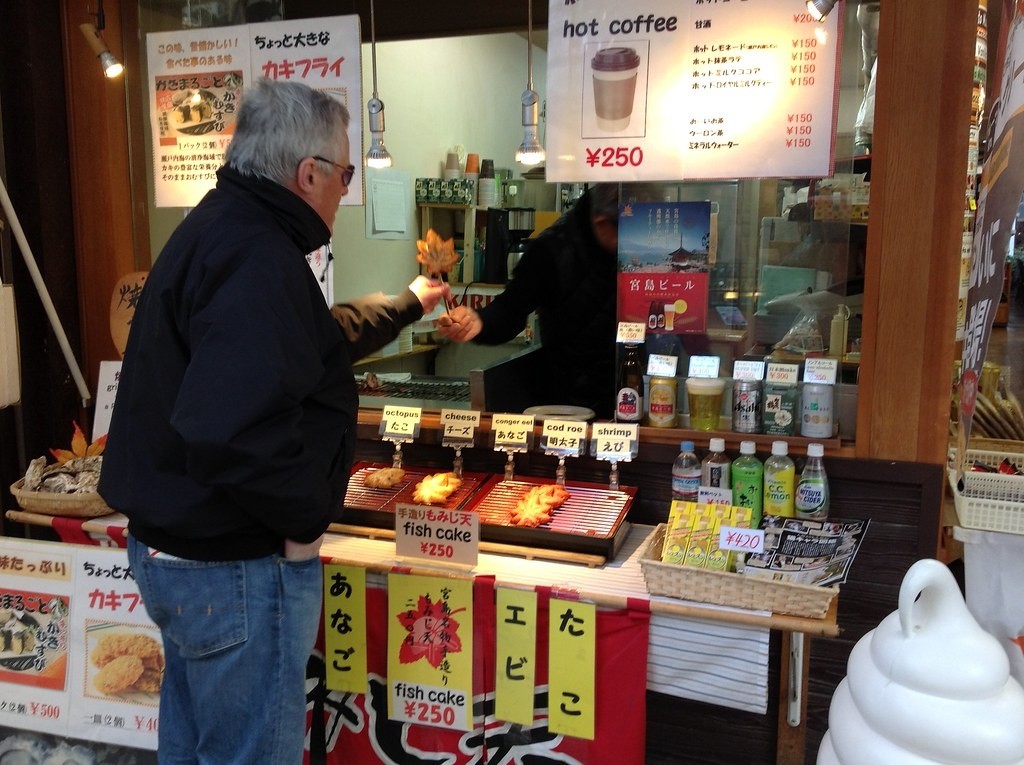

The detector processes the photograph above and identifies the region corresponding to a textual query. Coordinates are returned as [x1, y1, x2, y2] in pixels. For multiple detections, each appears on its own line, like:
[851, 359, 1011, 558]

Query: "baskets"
[10, 461, 115, 516]
[946, 448, 1023, 535]
[638, 523, 839, 621]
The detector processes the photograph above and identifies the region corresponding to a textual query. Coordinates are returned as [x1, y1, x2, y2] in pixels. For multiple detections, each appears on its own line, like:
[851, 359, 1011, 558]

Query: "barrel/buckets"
[454, 249, 481, 282]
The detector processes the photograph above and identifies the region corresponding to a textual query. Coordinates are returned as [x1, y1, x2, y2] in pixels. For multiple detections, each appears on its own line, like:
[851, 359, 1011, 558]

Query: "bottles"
[615, 343, 644, 426]
[731, 441, 763, 529]
[702, 437, 733, 491]
[830, 304, 850, 360]
[648, 299, 665, 330]
[795, 443, 829, 522]
[763, 441, 796, 519]
[671, 441, 701, 502]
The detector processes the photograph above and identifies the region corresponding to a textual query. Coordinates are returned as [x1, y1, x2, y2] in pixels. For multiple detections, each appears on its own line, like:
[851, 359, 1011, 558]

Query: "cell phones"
[715, 306, 748, 326]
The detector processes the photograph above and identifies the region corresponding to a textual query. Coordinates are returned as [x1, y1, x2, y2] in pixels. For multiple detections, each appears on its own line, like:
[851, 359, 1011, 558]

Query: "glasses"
[297, 155, 355, 186]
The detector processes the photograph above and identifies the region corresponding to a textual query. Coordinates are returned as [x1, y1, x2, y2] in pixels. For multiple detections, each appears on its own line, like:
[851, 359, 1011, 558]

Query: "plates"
[521, 173, 545, 180]
[522, 405, 595, 423]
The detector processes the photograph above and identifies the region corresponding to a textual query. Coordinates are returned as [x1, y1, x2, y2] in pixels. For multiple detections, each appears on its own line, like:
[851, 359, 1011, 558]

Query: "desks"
[418, 201, 489, 285]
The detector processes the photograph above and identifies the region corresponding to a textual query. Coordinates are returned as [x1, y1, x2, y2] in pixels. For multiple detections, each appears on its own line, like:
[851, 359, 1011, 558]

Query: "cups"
[415, 152, 525, 207]
[446, 263, 461, 283]
[685, 378, 725, 431]
[591, 47, 640, 133]
[664, 304, 675, 330]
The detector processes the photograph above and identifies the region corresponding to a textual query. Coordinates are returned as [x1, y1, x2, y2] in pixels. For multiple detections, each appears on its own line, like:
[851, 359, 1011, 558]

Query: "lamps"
[80, 0, 123, 79]
[803, 0, 841, 21]
[515, 0, 547, 164]
[366, 0, 394, 167]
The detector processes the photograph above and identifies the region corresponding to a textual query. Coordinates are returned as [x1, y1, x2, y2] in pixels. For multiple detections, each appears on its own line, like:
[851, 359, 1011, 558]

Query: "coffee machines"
[485, 204, 536, 286]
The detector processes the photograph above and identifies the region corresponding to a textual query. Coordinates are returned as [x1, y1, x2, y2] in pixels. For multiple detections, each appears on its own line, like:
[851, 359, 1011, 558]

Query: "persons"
[436, 183, 669, 362]
[96, 78, 451, 762]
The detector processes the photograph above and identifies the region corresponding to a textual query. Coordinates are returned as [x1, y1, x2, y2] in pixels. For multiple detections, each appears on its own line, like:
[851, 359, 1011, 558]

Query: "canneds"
[648, 376, 678, 428]
[800, 382, 833, 440]
[732, 380, 761, 433]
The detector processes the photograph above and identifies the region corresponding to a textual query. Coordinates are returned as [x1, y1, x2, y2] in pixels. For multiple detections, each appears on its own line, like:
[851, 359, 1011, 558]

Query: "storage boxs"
[812, 178, 873, 220]
[415, 178, 474, 205]
